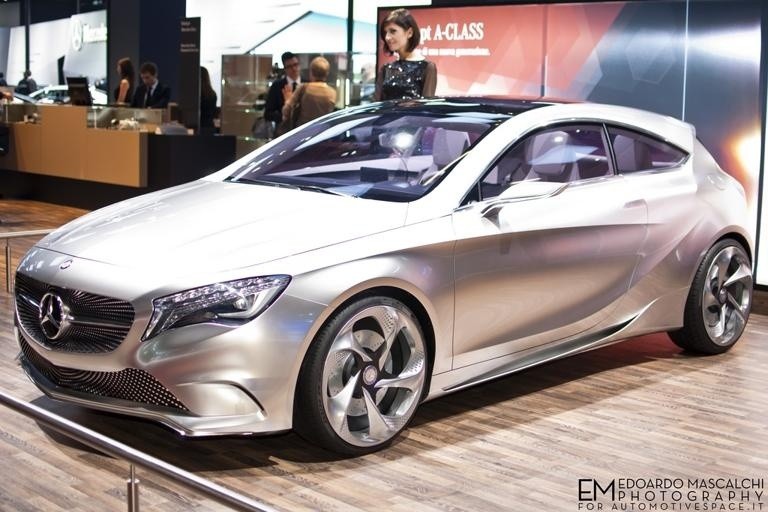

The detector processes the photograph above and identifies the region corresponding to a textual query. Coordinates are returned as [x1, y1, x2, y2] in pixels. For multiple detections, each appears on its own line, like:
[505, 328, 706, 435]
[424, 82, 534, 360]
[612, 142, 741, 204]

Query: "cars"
[12, 93, 755, 456]
[7, 82, 108, 110]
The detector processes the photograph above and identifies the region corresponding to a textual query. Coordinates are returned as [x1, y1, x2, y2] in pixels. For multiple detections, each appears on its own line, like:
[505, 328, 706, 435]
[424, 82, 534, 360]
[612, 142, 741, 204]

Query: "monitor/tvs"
[66, 76, 93, 106]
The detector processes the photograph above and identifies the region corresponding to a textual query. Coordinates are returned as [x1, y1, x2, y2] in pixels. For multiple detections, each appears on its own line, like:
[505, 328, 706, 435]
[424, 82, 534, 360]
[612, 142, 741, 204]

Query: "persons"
[282, 55, 339, 129]
[17, 70, 37, 98]
[0, 72, 7, 86]
[200, 65, 221, 131]
[113, 56, 134, 105]
[263, 50, 309, 140]
[131, 59, 172, 108]
[369, 7, 438, 155]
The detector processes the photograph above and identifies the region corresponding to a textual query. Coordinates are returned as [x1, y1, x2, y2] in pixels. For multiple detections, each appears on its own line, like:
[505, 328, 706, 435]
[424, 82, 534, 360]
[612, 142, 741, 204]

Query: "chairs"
[420, 128, 472, 195]
[503, 129, 579, 191]
[598, 134, 654, 177]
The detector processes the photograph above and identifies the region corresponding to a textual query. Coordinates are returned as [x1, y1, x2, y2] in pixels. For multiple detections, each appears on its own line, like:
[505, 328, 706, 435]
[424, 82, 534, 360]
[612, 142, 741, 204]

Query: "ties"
[291, 83, 298, 108]
[145, 87, 153, 108]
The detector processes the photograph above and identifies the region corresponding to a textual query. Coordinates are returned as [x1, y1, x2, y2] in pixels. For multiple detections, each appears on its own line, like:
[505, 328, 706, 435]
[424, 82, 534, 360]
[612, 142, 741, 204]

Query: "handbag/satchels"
[272, 102, 301, 140]
